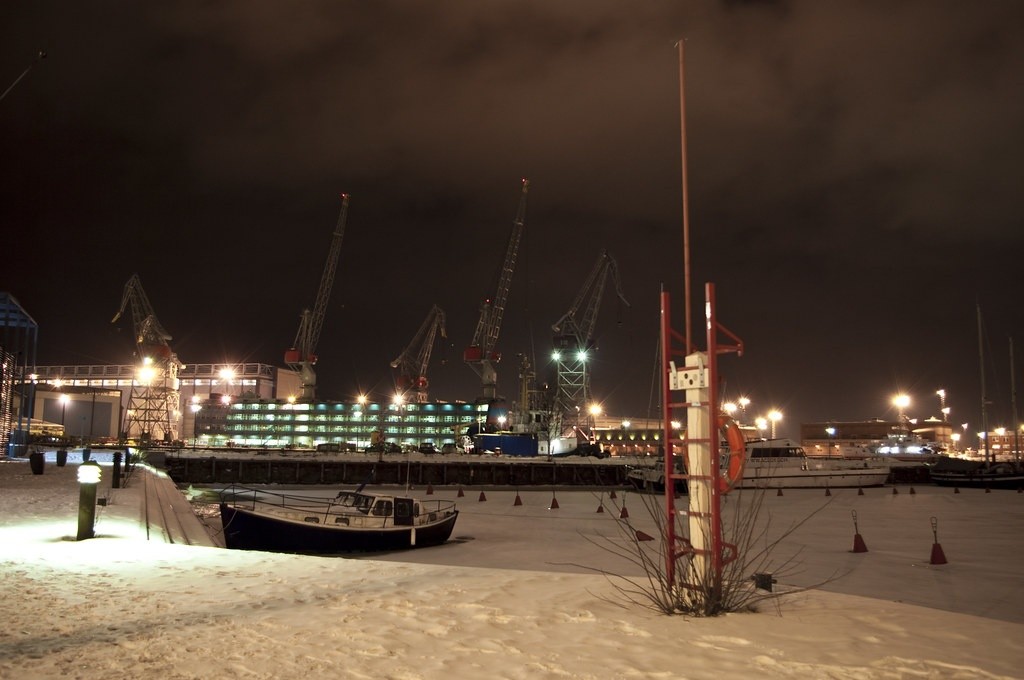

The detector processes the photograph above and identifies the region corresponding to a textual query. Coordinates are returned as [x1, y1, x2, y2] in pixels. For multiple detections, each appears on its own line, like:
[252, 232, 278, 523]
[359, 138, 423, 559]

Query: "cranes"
[552, 248, 632, 449]
[280, 192, 352, 366]
[112, 272, 187, 438]
[389, 303, 450, 391]
[462, 176, 532, 363]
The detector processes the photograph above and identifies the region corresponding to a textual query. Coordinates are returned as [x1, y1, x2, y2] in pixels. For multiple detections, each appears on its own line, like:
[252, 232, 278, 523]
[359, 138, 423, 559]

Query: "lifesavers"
[682, 409, 749, 499]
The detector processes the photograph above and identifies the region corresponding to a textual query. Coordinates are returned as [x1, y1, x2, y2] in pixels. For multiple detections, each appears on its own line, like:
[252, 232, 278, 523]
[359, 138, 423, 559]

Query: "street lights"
[937, 389, 948, 448]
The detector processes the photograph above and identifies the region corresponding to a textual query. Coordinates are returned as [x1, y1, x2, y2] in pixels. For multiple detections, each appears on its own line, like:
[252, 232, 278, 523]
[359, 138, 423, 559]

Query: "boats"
[929, 472, 1024, 489]
[218, 484, 460, 554]
[623, 435, 892, 490]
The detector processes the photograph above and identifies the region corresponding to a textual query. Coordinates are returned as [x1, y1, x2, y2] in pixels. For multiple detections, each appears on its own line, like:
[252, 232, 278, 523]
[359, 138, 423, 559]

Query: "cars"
[442, 443, 466, 456]
[370, 441, 418, 454]
[418, 442, 437, 453]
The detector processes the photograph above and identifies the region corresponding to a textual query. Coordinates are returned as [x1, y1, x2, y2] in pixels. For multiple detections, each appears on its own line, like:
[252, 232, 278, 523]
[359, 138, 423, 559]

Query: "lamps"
[76, 460, 103, 484]
